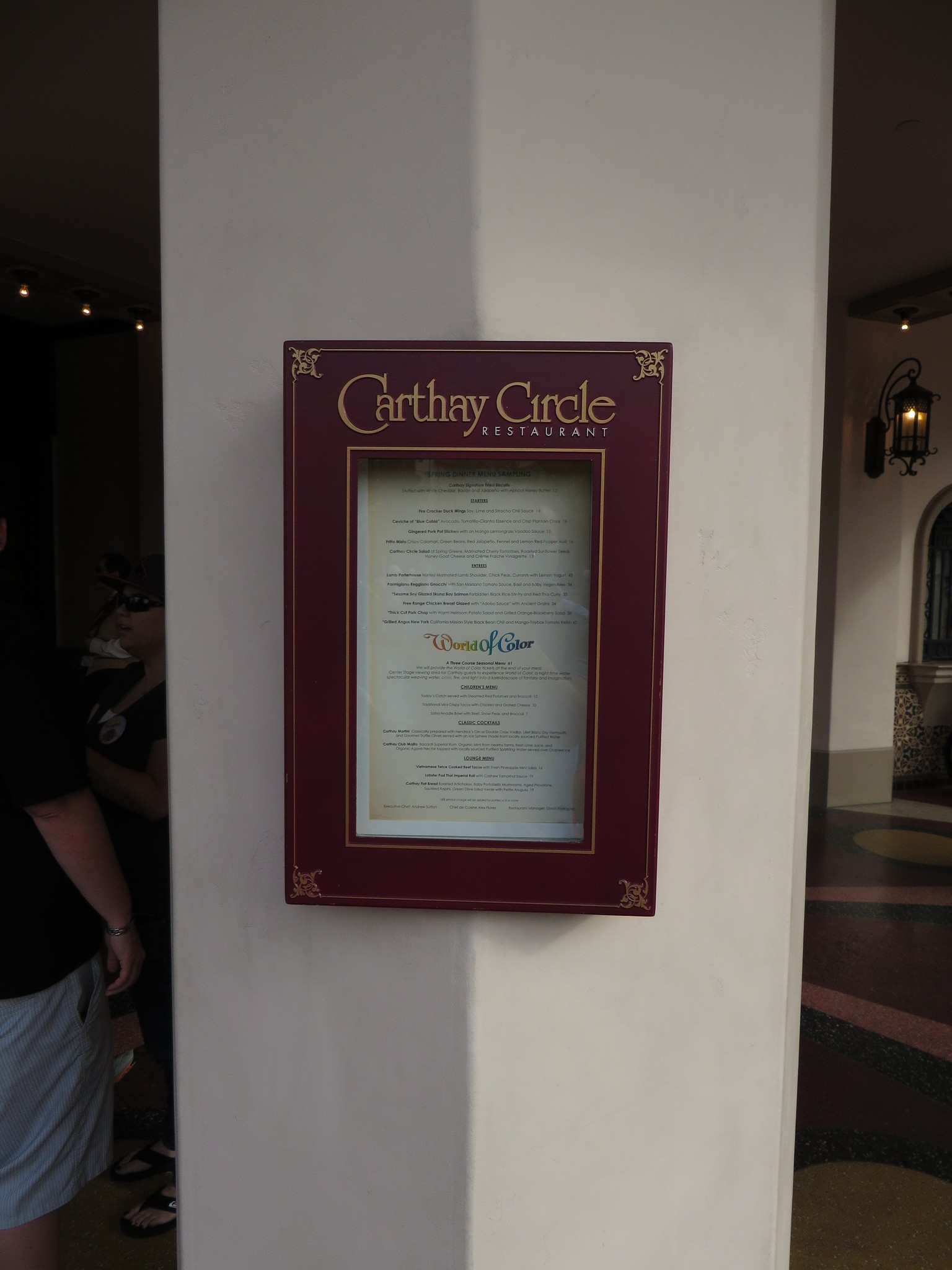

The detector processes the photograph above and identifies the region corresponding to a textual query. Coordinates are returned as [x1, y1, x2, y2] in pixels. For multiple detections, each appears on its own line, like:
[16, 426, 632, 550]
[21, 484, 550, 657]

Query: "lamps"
[863, 356, 942, 480]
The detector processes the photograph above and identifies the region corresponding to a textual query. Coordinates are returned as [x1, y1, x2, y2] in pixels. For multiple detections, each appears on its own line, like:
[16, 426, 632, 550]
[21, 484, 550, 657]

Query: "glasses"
[116, 593, 166, 613]
[95, 566, 107, 573]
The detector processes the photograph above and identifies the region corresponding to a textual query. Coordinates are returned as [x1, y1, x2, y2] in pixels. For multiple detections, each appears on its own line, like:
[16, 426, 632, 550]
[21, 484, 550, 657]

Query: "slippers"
[120, 1184, 176, 1239]
[110, 1140, 175, 1182]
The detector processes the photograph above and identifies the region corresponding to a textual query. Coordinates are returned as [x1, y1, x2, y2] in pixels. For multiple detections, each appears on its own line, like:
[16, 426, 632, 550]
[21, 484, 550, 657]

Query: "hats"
[94, 553, 165, 599]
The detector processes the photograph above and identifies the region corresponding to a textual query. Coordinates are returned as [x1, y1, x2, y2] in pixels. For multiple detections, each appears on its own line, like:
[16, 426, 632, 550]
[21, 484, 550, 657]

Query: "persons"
[1, 537, 179, 1270]
